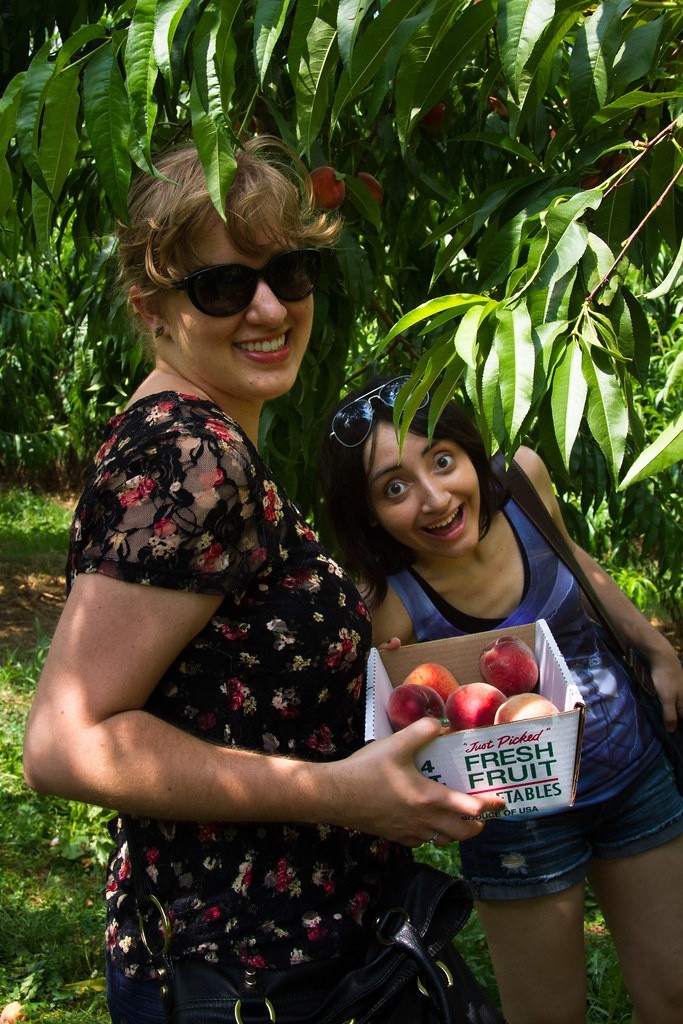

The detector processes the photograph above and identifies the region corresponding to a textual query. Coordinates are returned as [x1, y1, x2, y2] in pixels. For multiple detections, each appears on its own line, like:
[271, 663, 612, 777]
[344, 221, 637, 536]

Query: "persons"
[323, 380, 683, 1024]
[25, 140, 509, 1024]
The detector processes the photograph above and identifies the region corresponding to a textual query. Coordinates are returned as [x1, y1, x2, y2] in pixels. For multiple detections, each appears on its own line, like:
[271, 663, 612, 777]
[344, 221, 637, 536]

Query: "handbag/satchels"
[158, 855, 507, 1024]
[621, 645, 683, 795]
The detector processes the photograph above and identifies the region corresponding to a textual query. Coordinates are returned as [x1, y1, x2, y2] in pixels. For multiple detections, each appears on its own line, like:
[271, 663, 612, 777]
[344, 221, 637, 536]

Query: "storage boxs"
[365, 618, 586, 820]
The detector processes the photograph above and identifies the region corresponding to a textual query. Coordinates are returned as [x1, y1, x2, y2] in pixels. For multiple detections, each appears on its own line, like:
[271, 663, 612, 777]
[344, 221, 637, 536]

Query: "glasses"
[330, 374, 429, 447]
[162, 247, 323, 317]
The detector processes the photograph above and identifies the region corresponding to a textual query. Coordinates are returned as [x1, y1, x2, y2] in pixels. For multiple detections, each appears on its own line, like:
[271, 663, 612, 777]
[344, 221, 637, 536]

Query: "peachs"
[386, 633, 562, 734]
[418, 97, 632, 190]
[305, 166, 383, 211]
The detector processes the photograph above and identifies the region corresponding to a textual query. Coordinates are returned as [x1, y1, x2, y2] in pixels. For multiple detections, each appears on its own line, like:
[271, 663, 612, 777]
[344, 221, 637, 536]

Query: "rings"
[426, 832, 438, 843]
